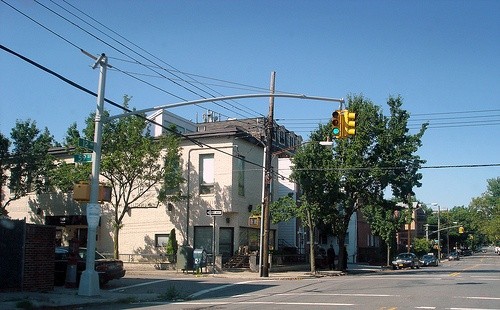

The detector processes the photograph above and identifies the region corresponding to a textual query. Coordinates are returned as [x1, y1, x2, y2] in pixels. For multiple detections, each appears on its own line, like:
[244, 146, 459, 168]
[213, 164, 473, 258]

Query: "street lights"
[408, 202, 437, 251]
[263, 141, 335, 276]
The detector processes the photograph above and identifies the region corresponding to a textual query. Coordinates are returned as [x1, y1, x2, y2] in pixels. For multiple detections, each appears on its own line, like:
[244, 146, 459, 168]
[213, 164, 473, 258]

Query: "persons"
[62, 238, 81, 288]
[327, 245, 336, 270]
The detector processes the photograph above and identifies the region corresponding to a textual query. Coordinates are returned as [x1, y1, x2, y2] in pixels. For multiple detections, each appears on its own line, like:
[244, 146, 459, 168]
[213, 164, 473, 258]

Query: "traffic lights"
[99, 185, 112, 203]
[331, 110, 341, 140]
[249, 217, 260, 227]
[343, 109, 356, 139]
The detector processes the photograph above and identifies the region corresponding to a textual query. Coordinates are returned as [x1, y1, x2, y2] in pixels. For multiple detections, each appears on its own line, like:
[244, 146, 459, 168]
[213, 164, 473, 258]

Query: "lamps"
[168, 203, 172, 211]
[248, 204, 252, 213]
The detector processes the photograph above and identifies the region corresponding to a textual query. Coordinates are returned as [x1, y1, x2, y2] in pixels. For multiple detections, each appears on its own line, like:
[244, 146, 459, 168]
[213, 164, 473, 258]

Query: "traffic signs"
[205, 209, 224, 217]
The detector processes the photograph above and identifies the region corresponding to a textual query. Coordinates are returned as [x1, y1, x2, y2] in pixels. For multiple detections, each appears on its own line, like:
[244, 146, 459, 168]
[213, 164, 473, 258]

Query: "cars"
[419, 253, 440, 266]
[391, 252, 421, 270]
[475, 247, 500, 255]
[55, 247, 126, 290]
[448, 247, 472, 261]
